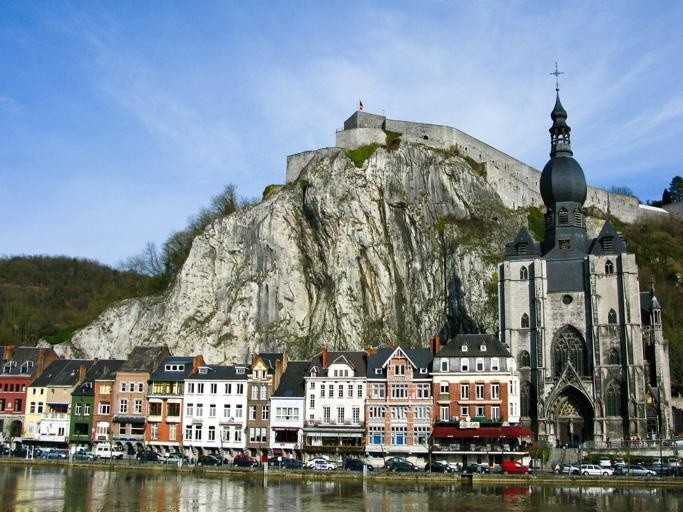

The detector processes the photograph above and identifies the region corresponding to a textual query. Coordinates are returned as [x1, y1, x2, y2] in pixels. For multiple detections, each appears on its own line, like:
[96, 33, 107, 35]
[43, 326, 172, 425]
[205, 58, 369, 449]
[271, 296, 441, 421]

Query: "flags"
[360, 101, 363, 111]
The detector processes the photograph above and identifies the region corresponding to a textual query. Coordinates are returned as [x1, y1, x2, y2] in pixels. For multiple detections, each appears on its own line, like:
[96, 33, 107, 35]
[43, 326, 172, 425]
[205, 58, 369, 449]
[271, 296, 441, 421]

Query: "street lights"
[645, 381, 665, 478]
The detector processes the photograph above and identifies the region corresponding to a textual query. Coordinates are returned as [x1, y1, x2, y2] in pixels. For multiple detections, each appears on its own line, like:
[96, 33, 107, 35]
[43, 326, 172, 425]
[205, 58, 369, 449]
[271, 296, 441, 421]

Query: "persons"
[632, 435, 640, 448]
